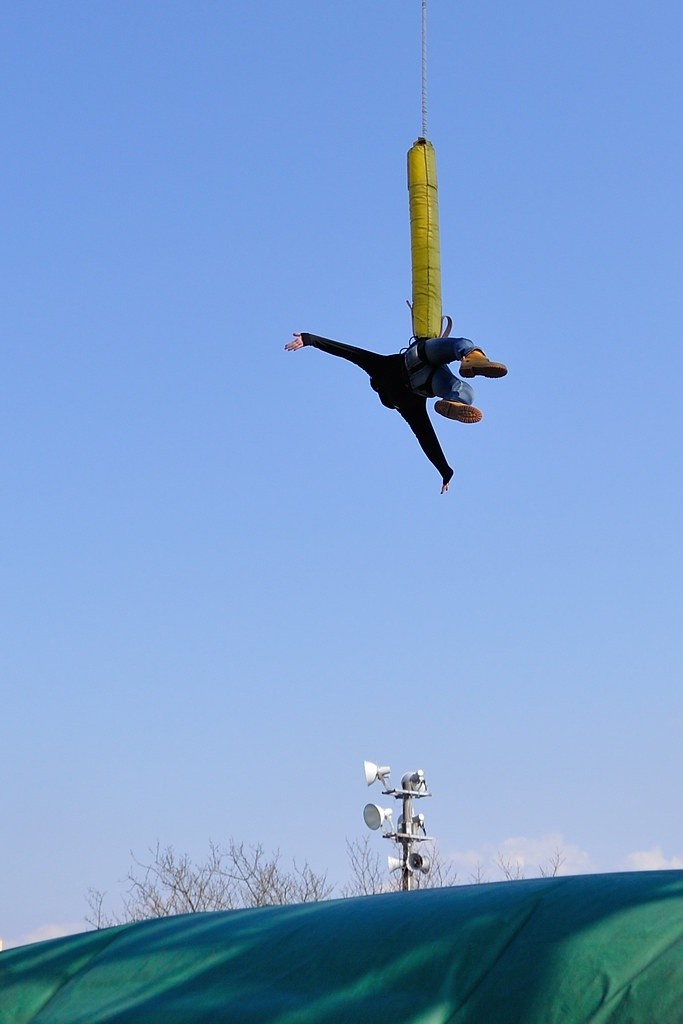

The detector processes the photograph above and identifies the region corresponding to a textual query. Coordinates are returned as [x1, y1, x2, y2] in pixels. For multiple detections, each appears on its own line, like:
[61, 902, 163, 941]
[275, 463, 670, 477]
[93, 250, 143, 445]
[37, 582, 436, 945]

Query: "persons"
[285, 330, 508, 494]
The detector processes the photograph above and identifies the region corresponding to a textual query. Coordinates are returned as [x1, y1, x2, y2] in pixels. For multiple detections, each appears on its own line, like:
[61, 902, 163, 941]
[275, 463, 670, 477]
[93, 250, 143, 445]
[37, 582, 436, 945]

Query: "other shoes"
[459, 350, 508, 378]
[434, 400, 482, 423]
